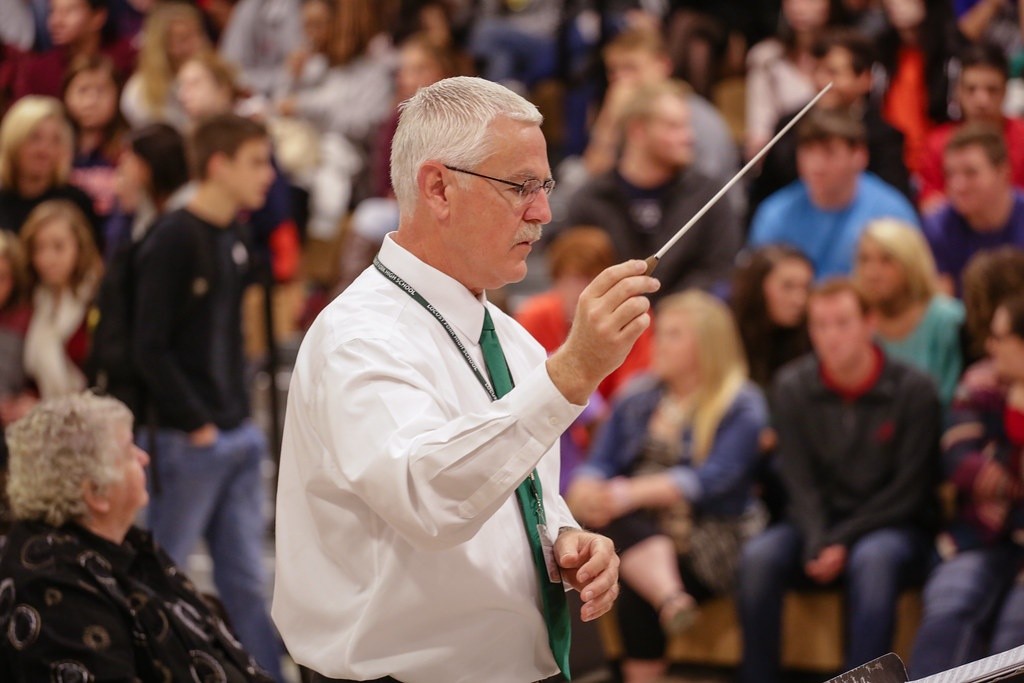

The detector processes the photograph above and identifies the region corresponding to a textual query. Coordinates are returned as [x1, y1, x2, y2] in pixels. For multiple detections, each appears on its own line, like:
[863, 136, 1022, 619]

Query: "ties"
[479, 307, 571, 682]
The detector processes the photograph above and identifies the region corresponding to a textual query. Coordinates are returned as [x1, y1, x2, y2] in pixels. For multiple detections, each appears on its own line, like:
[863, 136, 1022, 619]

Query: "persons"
[732, 280, 942, 683]
[912, 292, 1024, 683]
[133, 115, 283, 683]
[0, 390, 271, 683]
[0, 0, 1024, 423]
[569, 290, 769, 683]
[271, 76, 660, 683]
[748, 114, 917, 280]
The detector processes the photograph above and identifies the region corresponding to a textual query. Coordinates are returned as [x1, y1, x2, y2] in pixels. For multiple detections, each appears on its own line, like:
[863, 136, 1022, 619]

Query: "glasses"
[443, 164, 557, 204]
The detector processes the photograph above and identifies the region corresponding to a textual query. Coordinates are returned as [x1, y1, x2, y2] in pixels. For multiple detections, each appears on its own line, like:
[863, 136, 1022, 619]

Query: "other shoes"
[659, 591, 701, 632]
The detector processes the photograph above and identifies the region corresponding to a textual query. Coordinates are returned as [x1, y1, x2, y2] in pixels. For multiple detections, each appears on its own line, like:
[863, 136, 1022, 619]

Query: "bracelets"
[614, 478, 631, 508]
